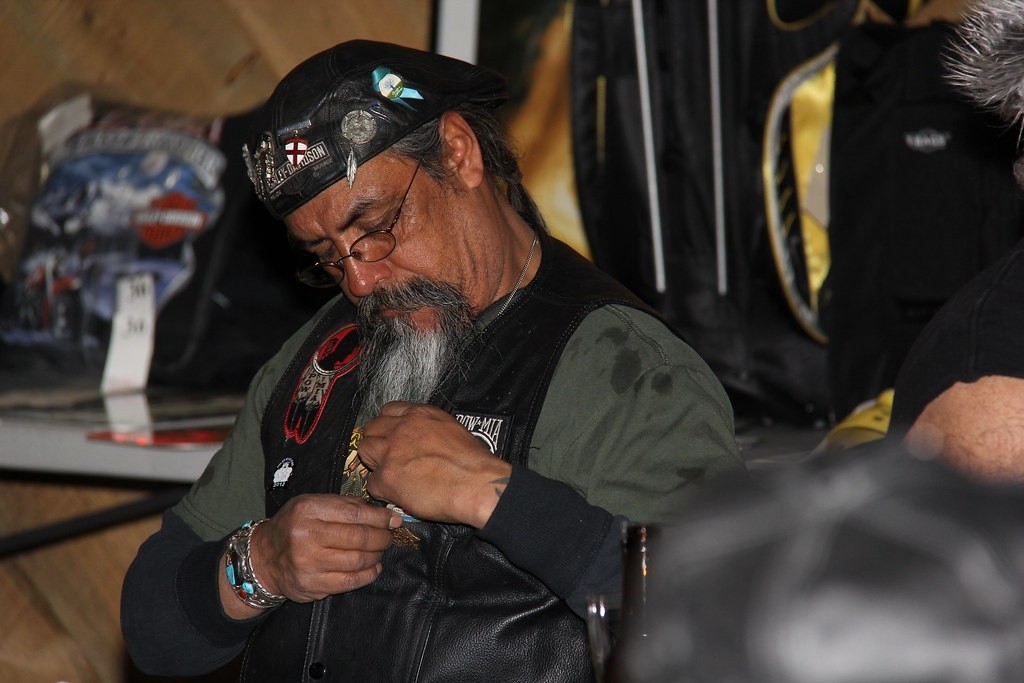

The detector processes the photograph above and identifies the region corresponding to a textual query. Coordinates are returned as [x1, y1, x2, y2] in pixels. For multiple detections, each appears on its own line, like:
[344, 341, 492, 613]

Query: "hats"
[243, 39, 510, 221]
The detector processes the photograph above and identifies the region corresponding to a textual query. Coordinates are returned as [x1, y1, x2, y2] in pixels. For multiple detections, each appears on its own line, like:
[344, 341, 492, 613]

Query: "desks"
[0, 417, 239, 563]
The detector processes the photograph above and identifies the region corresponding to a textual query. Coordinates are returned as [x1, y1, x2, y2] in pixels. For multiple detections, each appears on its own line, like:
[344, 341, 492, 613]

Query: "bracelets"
[224, 518, 287, 610]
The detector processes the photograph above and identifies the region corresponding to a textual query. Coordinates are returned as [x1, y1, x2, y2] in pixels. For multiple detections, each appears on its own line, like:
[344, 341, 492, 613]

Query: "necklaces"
[498, 233, 539, 316]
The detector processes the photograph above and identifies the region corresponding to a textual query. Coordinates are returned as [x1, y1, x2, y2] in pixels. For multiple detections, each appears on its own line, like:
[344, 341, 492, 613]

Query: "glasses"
[296, 157, 422, 289]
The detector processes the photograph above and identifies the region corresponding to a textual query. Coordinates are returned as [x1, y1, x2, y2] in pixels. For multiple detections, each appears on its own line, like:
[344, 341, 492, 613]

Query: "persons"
[120, 38, 748, 683]
[615, 0, 1023, 683]
